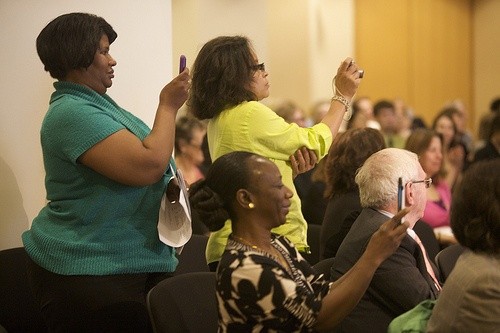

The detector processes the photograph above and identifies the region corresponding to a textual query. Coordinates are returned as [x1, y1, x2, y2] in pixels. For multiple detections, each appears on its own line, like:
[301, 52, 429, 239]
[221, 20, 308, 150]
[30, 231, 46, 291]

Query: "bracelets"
[330, 96, 351, 112]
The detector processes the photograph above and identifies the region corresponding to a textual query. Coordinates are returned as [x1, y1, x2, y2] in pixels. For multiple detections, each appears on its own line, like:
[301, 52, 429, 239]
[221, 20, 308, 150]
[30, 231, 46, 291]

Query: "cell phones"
[397, 176, 404, 227]
[179, 54, 187, 75]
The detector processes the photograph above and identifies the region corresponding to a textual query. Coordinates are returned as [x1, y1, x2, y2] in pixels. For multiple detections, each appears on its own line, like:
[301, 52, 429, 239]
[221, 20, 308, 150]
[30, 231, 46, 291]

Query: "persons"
[317, 128, 385, 260]
[276, 97, 500, 226]
[187, 37, 361, 271]
[172, 112, 207, 188]
[188, 151, 409, 333]
[329, 146, 444, 333]
[427, 157, 500, 333]
[21, 13, 191, 333]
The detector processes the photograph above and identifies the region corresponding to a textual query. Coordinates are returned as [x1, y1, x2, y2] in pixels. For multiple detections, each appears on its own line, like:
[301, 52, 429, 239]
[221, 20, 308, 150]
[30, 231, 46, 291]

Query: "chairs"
[0, 224, 460, 333]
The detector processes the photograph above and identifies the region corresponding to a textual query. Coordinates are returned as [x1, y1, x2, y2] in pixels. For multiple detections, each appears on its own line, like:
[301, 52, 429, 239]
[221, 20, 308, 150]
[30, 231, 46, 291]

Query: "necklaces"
[228, 233, 288, 269]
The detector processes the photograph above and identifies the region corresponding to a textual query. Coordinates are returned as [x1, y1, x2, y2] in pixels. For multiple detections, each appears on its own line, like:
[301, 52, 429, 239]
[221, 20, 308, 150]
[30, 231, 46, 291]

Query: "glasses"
[413, 178, 432, 188]
[249, 63, 265, 72]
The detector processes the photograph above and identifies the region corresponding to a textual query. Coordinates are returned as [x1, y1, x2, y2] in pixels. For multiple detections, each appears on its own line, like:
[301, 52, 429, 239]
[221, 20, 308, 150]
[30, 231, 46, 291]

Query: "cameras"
[339, 61, 364, 79]
